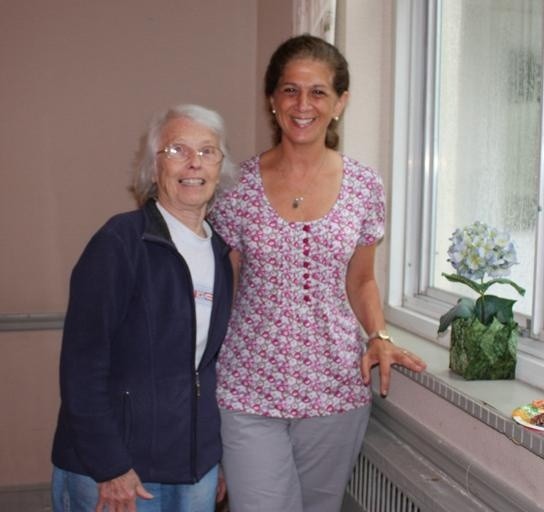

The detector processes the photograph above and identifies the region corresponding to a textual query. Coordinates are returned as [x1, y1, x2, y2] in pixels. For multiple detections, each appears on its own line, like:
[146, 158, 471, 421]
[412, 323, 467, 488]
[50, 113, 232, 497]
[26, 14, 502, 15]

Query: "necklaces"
[278, 149, 329, 210]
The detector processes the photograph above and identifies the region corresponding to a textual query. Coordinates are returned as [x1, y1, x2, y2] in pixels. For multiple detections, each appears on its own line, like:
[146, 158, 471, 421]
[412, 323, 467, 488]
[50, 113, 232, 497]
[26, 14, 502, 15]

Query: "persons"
[49, 101, 234, 511]
[201, 33, 426, 512]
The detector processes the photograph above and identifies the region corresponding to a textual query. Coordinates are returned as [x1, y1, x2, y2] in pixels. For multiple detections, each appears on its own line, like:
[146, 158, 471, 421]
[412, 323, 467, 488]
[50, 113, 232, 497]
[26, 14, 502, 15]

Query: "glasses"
[157, 142, 226, 165]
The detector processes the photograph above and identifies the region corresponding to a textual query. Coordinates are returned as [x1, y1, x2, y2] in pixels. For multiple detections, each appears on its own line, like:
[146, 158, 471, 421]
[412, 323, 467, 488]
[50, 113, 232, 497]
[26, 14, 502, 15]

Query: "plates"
[511, 399, 544, 432]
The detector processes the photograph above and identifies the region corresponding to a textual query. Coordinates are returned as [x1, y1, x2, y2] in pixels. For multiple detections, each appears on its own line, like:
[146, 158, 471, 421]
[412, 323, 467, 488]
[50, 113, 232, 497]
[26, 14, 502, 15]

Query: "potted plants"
[438, 295, 520, 381]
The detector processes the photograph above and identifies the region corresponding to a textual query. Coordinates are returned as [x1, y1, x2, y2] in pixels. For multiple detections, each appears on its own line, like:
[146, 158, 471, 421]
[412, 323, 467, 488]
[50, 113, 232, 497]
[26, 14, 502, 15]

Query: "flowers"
[441, 219, 526, 304]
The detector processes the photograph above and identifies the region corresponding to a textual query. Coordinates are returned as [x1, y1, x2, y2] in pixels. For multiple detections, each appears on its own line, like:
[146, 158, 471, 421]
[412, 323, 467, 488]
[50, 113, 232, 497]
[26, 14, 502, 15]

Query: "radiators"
[340, 410, 518, 512]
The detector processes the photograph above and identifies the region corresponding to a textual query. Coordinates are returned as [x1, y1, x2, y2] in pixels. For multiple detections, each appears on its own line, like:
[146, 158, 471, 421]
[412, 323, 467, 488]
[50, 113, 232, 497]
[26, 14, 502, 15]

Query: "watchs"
[367, 329, 393, 342]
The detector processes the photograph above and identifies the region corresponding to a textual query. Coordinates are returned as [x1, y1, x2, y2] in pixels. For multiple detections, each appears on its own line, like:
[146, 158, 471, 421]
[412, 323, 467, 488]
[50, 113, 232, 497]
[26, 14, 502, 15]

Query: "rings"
[401, 349, 407, 354]
